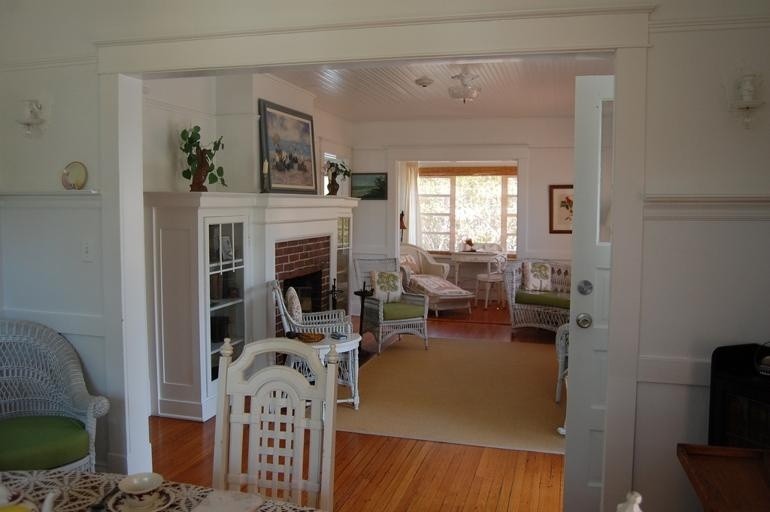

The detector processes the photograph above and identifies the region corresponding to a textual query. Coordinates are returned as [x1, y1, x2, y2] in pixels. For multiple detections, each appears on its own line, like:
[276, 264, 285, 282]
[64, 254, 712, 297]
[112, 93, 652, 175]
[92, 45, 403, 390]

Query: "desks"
[0, 465, 320, 512]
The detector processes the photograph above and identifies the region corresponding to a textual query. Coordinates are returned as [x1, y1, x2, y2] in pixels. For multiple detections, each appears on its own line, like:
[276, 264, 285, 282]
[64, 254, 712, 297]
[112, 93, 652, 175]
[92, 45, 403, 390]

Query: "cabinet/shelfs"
[144, 190, 252, 422]
[337, 198, 360, 319]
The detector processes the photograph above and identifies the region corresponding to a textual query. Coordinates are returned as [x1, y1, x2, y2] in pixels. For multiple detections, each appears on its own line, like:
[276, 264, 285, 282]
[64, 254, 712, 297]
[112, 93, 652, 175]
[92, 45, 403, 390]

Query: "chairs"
[271, 279, 362, 413]
[0, 319, 111, 474]
[209, 334, 340, 512]
[352, 243, 510, 356]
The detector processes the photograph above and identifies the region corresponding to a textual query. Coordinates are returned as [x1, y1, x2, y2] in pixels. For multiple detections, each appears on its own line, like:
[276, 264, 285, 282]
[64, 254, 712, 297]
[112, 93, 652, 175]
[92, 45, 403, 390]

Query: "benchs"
[500, 257, 573, 340]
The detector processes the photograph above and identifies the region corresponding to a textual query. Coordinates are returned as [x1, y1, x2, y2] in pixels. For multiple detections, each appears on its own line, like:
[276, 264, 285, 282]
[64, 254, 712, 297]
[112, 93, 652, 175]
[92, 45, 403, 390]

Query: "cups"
[22, 99, 42, 119]
[119, 472, 164, 509]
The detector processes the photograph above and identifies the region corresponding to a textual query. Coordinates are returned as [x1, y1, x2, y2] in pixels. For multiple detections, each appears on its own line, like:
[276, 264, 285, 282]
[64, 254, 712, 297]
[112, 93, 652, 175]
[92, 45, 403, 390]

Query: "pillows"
[522, 260, 552, 292]
[284, 285, 304, 326]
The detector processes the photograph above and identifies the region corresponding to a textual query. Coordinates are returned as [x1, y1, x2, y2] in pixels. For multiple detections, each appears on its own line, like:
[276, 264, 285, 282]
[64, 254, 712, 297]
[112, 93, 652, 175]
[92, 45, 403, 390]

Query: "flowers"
[321, 155, 351, 184]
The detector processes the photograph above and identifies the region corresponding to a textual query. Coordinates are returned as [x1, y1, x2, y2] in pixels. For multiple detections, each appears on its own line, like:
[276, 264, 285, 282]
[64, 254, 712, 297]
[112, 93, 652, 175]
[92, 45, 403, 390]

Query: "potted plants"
[178, 125, 228, 192]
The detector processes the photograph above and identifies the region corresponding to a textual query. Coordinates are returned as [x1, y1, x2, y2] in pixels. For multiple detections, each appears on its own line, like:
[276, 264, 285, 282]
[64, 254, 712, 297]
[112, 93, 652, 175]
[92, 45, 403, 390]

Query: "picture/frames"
[548, 184, 573, 235]
[258, 97, 318, 195]
[350, 172, 388, 200]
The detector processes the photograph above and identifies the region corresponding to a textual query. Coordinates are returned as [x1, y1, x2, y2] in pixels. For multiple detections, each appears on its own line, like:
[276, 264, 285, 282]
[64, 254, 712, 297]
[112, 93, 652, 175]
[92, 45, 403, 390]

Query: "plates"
[16, 118, 47, 126]
[106, 488, 176, 511]
[61, 162, 88, 190]
[1, 488, 24, 505]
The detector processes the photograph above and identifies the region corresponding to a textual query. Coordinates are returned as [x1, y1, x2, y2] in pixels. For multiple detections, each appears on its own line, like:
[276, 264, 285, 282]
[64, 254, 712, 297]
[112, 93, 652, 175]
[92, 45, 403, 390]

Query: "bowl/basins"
[299, 333, 324, 342]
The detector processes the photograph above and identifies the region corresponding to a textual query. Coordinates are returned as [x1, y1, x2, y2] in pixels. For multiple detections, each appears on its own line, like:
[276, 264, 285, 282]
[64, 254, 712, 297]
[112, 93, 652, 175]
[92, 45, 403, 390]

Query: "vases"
[326, 175, 340, 195]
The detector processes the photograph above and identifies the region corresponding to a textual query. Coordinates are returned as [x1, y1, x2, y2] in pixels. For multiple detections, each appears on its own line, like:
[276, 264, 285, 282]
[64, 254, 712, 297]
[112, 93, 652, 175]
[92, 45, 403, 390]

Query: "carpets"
[305, 334, 567, 455]
